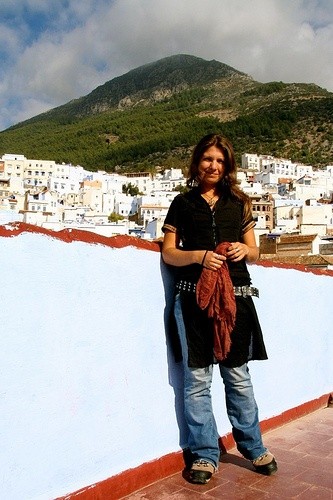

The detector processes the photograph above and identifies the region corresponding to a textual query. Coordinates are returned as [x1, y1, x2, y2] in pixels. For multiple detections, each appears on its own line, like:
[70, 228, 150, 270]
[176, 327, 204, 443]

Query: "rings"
[235, 248, 240, 252]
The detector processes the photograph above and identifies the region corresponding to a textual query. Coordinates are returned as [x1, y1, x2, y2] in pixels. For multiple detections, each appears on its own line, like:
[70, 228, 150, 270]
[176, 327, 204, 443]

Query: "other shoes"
[252, 449, 278, 476]
[189, 460, 214, 484]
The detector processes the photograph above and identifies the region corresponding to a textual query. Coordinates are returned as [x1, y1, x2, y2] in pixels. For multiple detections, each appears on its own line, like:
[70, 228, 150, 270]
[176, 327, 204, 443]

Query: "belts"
[177, 278, 260, 298]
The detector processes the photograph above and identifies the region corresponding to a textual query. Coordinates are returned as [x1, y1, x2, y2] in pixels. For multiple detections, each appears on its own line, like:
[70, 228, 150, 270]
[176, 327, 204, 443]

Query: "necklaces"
[204, 194, 216, 205]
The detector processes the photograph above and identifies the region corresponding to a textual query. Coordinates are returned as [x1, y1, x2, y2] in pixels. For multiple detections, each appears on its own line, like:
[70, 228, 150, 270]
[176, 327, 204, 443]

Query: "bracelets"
[201, 250, 208, 266]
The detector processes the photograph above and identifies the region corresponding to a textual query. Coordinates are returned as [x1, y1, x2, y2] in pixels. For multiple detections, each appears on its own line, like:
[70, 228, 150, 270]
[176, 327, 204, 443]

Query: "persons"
[161, 133, 278, 484]
